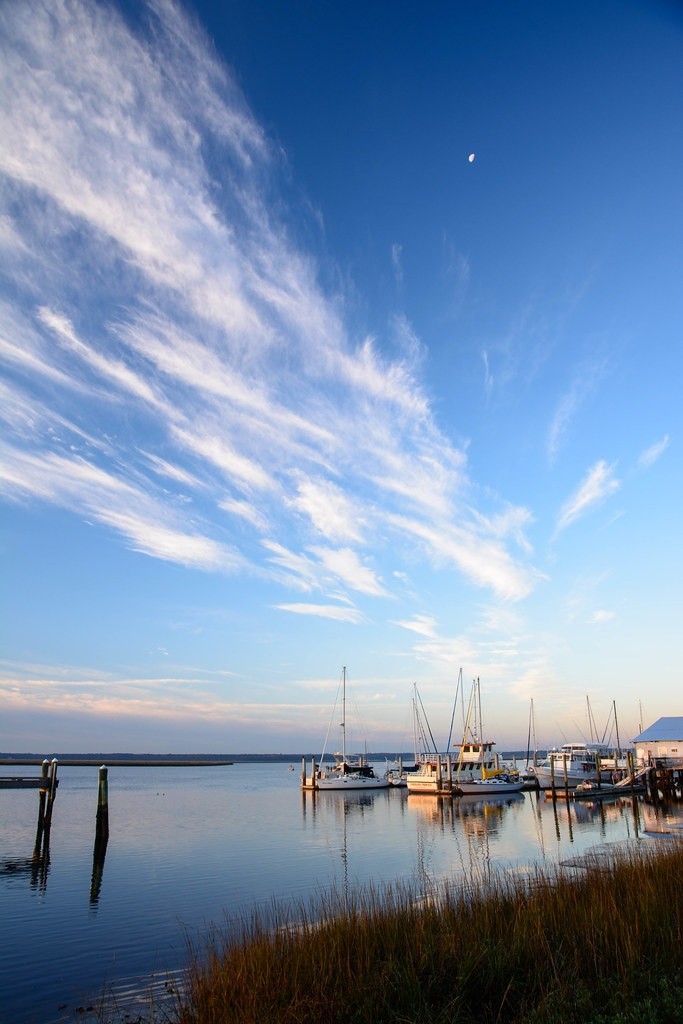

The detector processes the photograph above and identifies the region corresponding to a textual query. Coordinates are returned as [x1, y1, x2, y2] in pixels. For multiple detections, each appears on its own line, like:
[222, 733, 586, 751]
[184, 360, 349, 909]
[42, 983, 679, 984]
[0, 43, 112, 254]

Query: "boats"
[529, 741, 625, 787]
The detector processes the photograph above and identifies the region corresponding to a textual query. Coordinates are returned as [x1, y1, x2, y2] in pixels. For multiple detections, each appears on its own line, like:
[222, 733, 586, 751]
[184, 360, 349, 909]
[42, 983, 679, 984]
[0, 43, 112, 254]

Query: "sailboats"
[382, 667, 526, 795]
[302, 665, 390, 790]
[501, 696, 637, 784]
